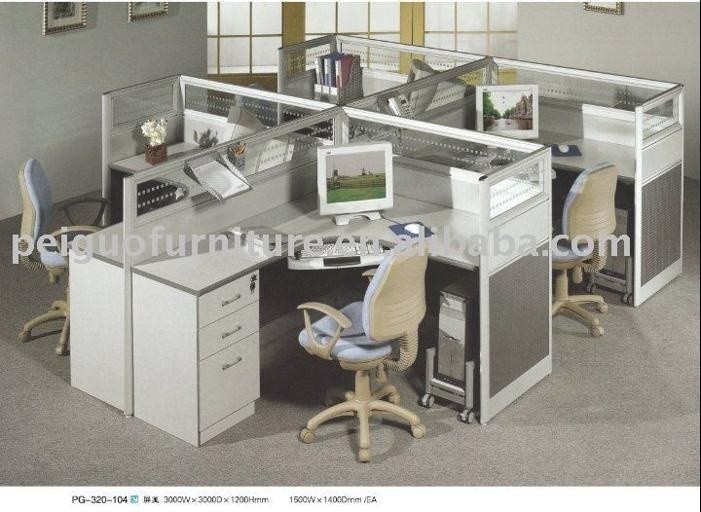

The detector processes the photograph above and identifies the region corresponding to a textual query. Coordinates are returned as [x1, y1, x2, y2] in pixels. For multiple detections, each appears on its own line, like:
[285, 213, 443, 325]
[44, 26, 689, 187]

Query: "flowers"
[140, 118, 168, 145]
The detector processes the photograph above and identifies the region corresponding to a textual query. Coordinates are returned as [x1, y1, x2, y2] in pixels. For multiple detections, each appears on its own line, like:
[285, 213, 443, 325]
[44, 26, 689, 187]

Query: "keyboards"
[298, 242, 385, 260]
[468, 166, 557, 181]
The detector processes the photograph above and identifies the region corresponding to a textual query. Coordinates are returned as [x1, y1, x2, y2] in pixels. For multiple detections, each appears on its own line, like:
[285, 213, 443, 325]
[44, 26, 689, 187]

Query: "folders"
[315, 53, 353, 88]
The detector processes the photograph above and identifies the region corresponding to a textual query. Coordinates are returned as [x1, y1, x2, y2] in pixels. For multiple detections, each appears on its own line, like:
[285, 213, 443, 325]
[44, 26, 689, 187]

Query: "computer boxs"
[434, 279, 479, 387]
[597, 195, 633, 285]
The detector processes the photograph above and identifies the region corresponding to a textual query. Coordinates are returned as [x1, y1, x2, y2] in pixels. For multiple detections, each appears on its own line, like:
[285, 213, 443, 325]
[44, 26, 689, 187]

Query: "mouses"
[559, 145, 570, 154]
[404, 223, 424, 235]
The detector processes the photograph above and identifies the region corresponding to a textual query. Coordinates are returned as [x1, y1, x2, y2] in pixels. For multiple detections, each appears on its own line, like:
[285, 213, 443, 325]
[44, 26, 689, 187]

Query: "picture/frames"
[128, 3, 173, 22]
[42, 3, 88, 36]
[584, 2, 623, 16]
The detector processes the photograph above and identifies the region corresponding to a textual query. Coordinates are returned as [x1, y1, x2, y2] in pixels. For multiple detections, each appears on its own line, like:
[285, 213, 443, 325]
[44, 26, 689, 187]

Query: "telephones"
[388, 93, 414, 119]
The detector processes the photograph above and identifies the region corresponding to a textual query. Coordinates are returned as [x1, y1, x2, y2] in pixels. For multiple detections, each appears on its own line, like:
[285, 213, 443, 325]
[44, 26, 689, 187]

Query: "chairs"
[18, 156, 113, 356]
[297, 236, 432, 464]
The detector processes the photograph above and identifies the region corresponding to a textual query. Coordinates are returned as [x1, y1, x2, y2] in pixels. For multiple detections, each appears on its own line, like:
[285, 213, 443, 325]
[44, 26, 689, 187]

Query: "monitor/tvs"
[475, 84, 540, 149]
[404, 59, 467, 116]
[317, 141, 393, 226]
[223, 106, 295, 177]
